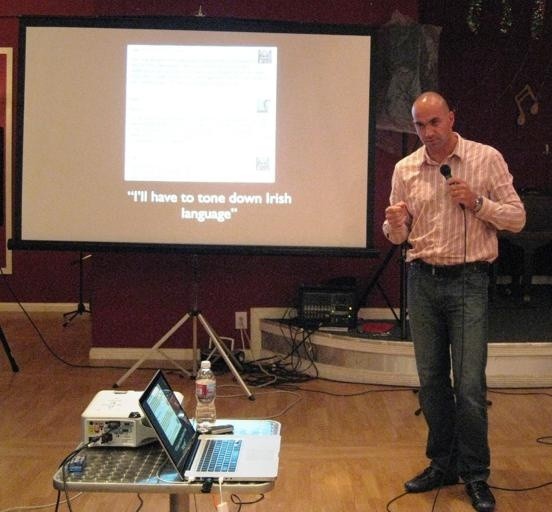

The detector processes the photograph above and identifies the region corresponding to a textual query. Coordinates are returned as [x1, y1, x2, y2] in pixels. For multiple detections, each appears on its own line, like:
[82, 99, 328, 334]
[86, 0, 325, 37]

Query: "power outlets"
[235, 311, 248, 330]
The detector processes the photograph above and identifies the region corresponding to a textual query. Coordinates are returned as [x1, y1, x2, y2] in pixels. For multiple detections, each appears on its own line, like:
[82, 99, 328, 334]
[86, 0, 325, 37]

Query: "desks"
[51, 416, 282, 511]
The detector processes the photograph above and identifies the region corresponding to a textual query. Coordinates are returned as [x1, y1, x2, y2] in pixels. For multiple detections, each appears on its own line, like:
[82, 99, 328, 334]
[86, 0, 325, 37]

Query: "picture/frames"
[0, 46, 12, 275]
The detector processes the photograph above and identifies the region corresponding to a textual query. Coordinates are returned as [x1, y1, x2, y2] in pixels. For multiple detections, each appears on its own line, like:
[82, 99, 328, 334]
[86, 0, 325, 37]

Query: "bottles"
[195, 360, 217, 434]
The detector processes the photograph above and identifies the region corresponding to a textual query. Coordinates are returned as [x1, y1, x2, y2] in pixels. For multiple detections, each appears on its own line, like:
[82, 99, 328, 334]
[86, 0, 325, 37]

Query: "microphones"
[440, 164, 465, 210]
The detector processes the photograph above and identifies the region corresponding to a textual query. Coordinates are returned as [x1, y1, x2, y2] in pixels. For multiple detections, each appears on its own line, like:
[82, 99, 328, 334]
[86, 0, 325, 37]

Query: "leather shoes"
[465, 480, 495, 511]
[404, 465, 459, 493]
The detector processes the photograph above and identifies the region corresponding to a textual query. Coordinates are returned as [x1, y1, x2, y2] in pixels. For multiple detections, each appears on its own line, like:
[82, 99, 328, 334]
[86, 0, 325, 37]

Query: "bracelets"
[473, 195, 484, 212]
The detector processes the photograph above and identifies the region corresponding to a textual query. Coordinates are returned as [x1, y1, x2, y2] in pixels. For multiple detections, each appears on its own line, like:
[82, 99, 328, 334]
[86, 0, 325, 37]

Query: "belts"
[411, 258, 489, 276]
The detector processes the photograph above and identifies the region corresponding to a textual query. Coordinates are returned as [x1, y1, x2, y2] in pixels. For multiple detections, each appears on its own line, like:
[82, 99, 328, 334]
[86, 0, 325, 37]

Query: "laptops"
[138, 369, 281, 481]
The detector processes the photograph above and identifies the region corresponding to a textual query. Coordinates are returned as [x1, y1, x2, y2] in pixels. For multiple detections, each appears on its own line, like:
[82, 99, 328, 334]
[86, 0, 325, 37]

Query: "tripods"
[63, 250, 93, 328]
[113, 253, 255, 401]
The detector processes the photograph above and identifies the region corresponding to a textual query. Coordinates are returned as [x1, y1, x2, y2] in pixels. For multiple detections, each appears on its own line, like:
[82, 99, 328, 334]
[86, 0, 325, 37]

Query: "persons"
[382, 91, 530, 512]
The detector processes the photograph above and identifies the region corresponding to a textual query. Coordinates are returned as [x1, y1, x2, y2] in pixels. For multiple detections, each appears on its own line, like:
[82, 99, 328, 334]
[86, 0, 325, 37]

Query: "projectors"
[80, 389, 184, 448]
